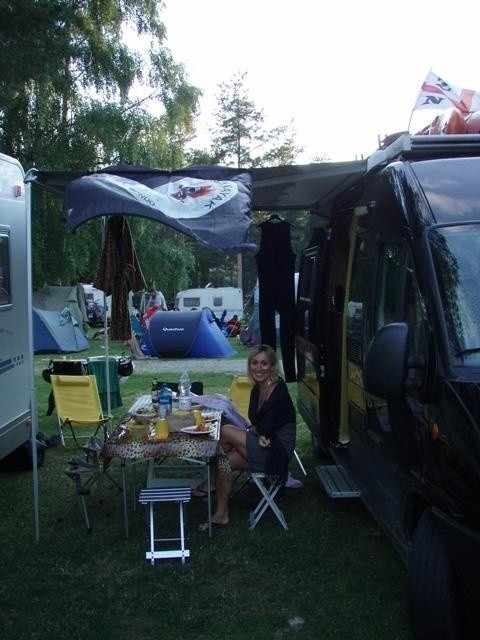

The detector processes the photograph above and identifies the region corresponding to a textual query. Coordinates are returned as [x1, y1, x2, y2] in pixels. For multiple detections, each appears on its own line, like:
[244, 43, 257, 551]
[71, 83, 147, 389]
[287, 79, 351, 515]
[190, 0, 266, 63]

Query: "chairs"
[157, 381, 203, 465]
[50, 374, 112, 449]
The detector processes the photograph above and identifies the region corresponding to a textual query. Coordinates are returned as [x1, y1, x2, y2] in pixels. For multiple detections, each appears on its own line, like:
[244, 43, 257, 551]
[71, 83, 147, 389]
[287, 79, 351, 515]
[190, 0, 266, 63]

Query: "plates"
[195, 413, 218, 423]
[180, 425, 217, 435]
[133, 407, 157, 418]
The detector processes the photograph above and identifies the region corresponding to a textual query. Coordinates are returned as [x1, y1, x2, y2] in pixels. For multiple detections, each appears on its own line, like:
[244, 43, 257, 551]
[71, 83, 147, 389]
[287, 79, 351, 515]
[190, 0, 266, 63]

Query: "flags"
[414, 72, 480, 118]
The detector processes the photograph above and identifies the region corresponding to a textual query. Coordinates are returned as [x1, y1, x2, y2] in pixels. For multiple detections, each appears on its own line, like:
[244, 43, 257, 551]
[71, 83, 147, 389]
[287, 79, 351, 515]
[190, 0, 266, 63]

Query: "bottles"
[151, 377, 172, 418]
[179, 368, 191, 411]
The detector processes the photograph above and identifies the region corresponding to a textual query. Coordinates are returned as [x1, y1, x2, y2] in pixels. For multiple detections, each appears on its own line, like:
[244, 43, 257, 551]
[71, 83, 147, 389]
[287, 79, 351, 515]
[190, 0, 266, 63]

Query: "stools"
[247, 471, 289, 531]
[139, 485, 192, 566]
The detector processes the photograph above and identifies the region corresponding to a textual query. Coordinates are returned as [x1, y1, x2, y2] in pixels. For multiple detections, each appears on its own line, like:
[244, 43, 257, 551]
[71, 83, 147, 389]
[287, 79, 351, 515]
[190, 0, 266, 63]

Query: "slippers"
[188, 485, 215, 498]
[197, 519, 229, 532]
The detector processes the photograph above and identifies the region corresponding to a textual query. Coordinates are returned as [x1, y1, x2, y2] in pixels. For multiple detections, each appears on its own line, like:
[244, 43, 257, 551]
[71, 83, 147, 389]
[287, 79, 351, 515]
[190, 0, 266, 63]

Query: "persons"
[149, 288, 168, 312]
[191, 345, 295, 532]
[227, 315, 241, 337]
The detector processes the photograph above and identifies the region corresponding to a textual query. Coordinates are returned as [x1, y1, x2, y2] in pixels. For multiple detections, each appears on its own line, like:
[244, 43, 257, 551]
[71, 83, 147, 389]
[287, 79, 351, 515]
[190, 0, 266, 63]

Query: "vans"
[247, 134, 479, 640]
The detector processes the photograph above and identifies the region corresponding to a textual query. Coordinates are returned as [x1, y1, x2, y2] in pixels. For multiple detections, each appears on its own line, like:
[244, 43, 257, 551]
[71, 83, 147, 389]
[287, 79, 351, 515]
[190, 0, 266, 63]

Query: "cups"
[154, 418, 170, 441]
